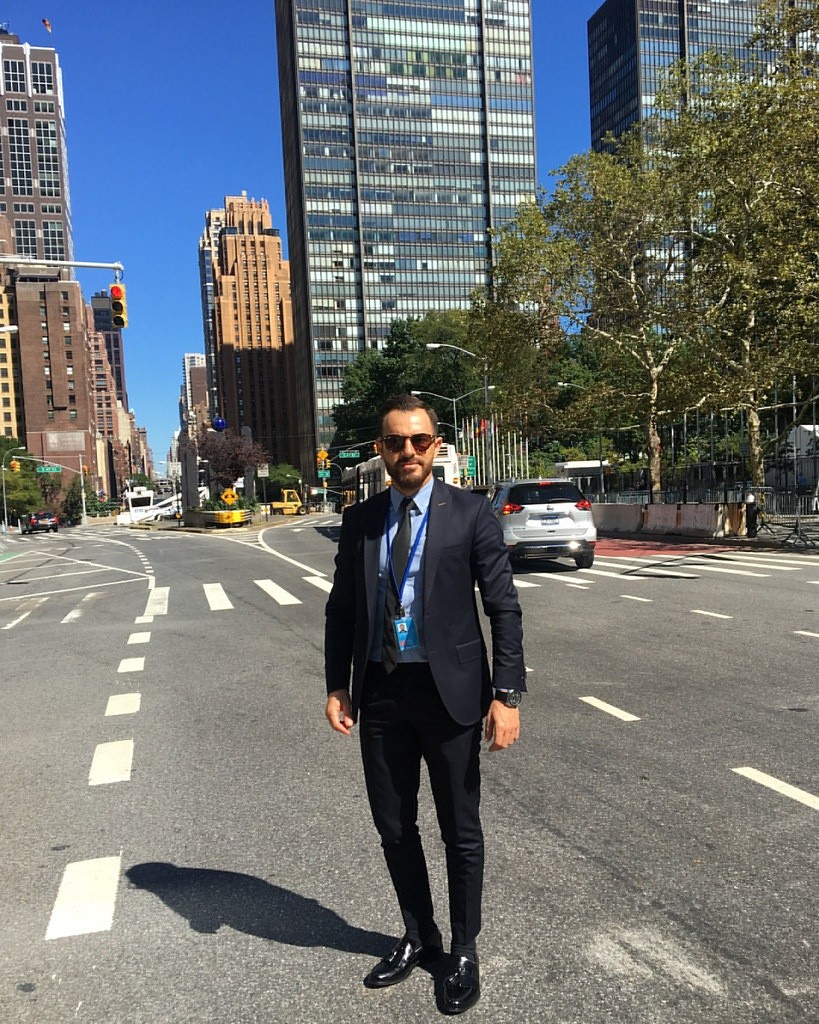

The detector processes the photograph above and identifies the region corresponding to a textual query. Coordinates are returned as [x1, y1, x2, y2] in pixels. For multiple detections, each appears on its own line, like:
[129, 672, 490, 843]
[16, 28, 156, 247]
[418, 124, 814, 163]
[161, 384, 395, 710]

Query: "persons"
[398, 624, 405, 633]
[322, 390, 527, 1015]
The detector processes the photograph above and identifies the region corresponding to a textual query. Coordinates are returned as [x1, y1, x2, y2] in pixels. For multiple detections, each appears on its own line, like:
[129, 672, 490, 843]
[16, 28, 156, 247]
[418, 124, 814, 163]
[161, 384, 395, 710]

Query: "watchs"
[494, 685, 522, 709]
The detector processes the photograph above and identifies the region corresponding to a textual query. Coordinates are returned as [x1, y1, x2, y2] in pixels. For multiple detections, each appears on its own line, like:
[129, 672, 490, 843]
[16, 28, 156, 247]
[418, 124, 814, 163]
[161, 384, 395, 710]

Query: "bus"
[340, 441, 462, 511]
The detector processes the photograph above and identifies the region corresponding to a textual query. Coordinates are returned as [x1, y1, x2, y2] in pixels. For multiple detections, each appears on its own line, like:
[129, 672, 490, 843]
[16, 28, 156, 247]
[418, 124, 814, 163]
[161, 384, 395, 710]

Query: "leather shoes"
[439, 950, 481, 1014]
[365, 924, 444, 989]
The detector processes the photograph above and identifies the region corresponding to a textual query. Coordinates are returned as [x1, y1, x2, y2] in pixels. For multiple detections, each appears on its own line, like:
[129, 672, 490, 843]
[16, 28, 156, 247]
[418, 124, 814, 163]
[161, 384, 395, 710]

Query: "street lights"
[556, 381, 605, 501]
[408, 341, 498, 488]
[2, 445, 27, 530]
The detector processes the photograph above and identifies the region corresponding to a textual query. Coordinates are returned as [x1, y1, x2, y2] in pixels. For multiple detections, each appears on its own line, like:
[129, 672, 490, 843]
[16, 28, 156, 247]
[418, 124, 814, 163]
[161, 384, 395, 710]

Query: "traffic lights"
[107, 282, 128, 330]
[11, 459, 22, 477]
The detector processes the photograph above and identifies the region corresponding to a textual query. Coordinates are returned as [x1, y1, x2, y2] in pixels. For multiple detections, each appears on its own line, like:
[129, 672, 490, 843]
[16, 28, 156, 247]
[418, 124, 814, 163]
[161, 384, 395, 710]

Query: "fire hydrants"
[744, 493, 760, 538]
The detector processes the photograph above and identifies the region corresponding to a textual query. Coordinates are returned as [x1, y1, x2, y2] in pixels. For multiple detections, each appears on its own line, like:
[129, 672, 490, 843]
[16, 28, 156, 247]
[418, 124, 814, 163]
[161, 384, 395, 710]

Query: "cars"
[485, 477, 597, 575]
[21, 511, 60, 535]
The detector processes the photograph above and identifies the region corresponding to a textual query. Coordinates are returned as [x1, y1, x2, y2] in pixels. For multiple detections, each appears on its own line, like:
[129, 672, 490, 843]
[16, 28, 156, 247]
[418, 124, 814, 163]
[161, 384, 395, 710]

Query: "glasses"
[379, 434, 438, 454]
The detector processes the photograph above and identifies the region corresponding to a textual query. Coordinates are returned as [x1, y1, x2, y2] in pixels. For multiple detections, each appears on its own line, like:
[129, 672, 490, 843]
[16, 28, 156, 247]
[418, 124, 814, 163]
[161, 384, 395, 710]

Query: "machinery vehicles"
[270, 488, 309, 516]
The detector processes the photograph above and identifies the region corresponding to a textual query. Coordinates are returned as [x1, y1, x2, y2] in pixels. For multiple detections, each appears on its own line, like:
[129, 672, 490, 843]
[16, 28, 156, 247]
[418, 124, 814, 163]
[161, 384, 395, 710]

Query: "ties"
[381, 496, 413, 677]
